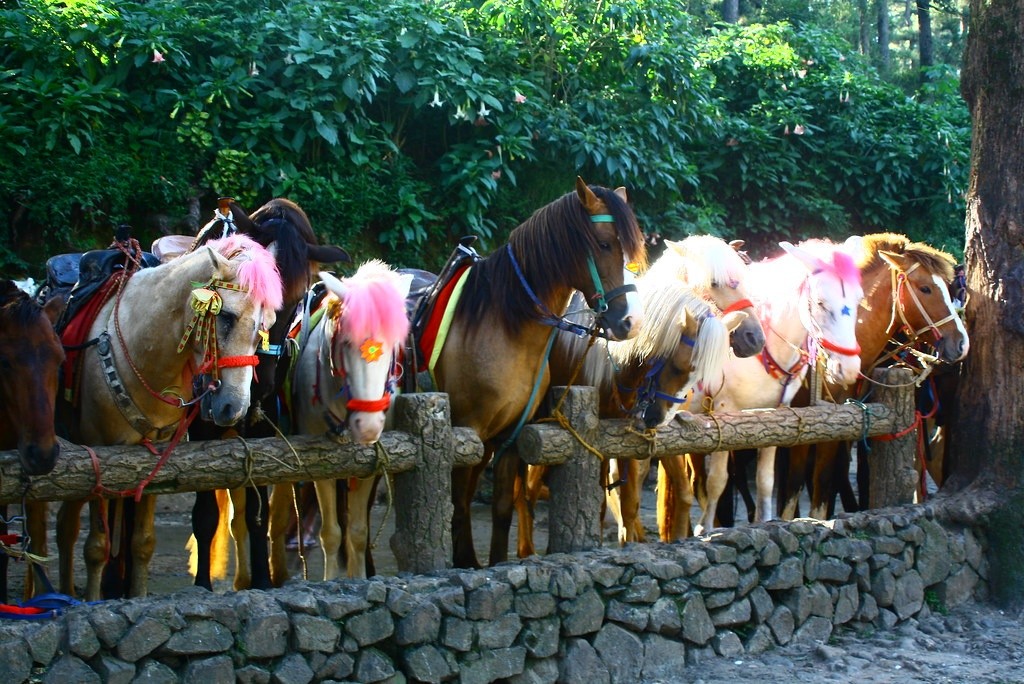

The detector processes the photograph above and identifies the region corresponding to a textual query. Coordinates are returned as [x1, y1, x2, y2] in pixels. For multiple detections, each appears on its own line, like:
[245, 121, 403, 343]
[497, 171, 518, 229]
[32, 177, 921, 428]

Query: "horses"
[0, 175, 972, 605]
